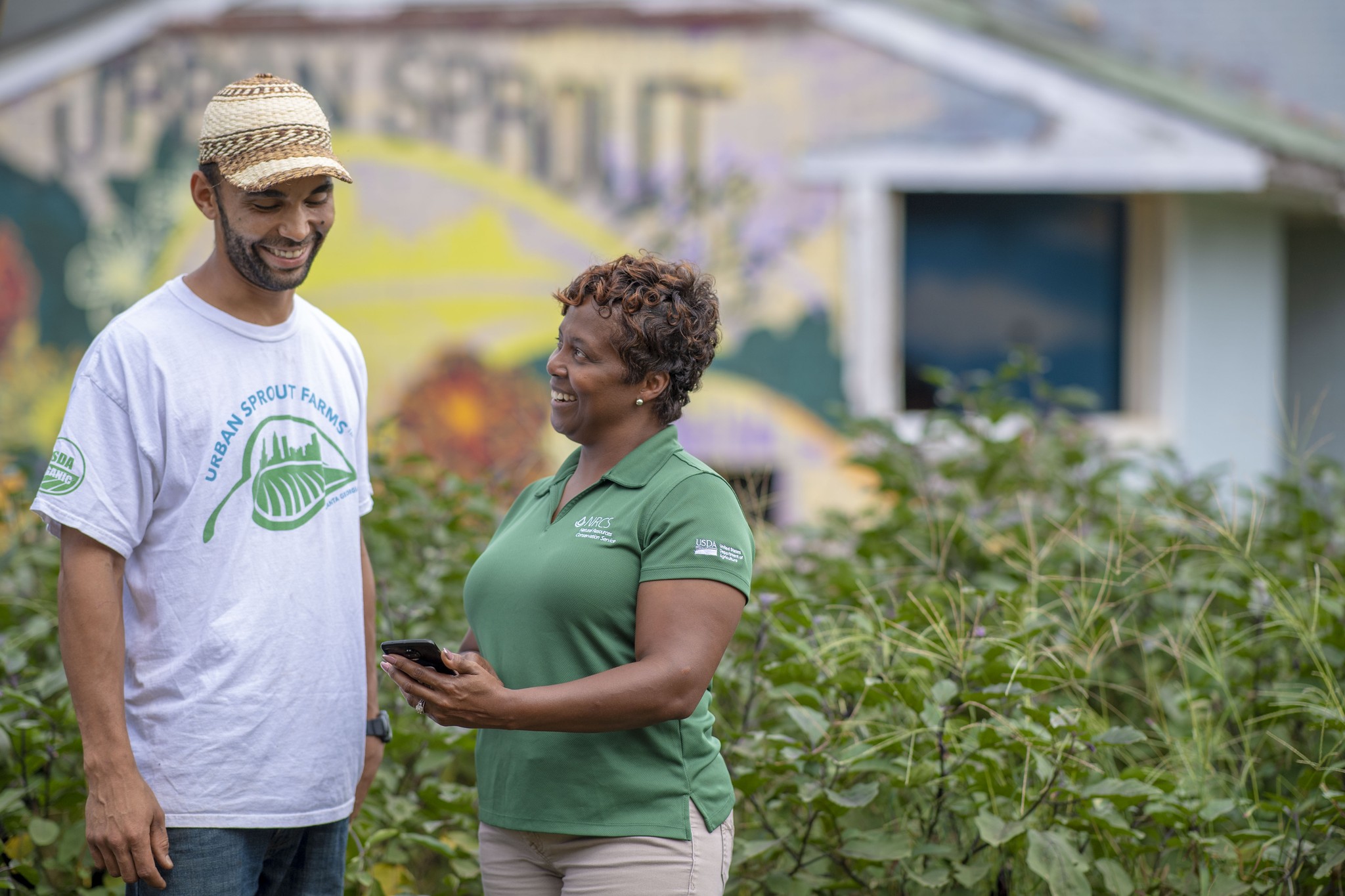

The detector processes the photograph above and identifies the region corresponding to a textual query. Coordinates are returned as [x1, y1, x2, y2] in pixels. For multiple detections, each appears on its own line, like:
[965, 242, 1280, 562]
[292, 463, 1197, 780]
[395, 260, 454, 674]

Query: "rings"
[415, 698, 428, 715]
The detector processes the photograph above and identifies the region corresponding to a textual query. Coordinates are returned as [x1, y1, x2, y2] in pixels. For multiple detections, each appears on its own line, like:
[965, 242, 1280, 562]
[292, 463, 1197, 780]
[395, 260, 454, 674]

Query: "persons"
[378, 253, 757, 896]
[27, 73, 393, 896]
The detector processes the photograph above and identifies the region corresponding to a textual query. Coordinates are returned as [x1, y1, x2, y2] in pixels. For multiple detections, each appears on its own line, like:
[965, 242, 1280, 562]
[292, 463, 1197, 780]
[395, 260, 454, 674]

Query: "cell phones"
[381, 638, 457, 690]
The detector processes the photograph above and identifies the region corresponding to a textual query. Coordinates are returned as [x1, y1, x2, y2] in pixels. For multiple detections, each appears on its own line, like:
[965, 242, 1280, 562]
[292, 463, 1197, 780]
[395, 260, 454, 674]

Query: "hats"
[198, 72, 352, 192]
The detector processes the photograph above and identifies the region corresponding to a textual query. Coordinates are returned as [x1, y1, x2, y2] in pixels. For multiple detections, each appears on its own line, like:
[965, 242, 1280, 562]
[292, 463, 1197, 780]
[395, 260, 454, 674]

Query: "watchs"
[365, 709, 392, 742]
